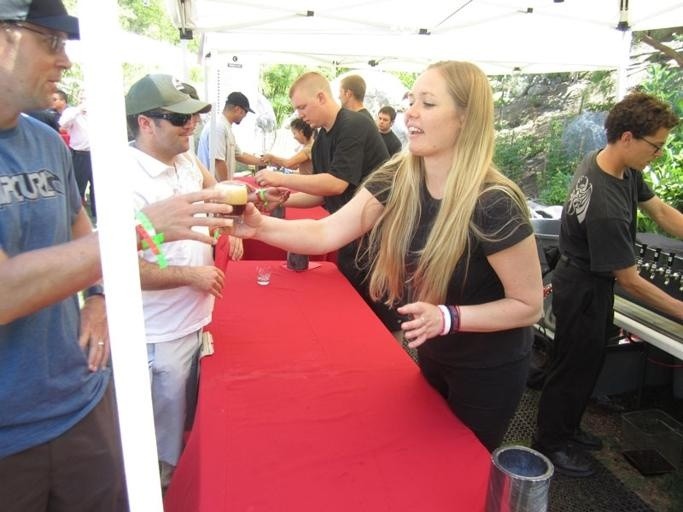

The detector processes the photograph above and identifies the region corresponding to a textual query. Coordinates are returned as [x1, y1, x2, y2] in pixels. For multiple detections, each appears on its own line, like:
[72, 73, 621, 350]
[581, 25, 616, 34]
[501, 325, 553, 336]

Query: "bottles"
[286, 251, 309, 269]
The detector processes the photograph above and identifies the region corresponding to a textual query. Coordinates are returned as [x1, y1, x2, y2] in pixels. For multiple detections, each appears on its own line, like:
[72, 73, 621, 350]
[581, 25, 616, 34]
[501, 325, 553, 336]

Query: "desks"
[232, 175, 340, 267]
[163, 261, 493, 512]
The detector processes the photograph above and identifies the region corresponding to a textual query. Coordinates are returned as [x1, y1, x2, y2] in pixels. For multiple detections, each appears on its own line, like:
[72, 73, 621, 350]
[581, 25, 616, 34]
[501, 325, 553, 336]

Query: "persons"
[0, 1, 290, 511]
[532, 94, 683, 477]
[211, 60, 542, 450]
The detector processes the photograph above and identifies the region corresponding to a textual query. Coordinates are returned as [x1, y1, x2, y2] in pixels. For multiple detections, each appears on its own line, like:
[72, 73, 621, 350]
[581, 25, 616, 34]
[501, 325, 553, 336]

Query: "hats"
[227, 91, 255, 114]
[125, 72, 212, 119]
[0, 0, 81, 40]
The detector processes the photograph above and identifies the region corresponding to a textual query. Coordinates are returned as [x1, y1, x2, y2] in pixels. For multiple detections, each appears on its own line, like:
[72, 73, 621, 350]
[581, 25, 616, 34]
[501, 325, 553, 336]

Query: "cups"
[215, 181, 246, 218]
[255, 266, 271, 286]
[484, 444, 552, 511]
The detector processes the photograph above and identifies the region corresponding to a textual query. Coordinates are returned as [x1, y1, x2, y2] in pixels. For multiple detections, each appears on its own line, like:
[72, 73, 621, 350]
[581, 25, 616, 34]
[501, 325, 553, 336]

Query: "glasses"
[631, 131, 662, 155]
[6, 21, 67, 54]
[142, 111, 192, 128]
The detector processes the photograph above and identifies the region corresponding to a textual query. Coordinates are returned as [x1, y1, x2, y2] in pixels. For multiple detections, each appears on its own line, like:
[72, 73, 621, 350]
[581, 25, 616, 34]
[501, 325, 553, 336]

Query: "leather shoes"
[530, 421, 606, 478]
